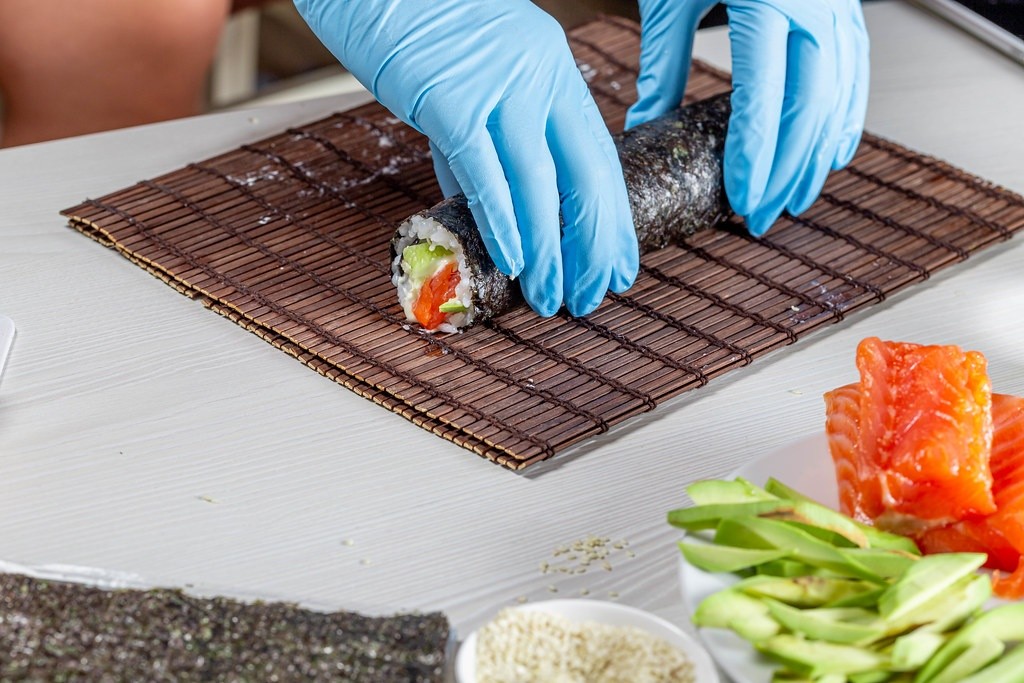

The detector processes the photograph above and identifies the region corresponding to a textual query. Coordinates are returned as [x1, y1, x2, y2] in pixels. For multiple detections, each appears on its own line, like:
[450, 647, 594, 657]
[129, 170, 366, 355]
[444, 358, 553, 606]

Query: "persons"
[0, 0, 870, 318]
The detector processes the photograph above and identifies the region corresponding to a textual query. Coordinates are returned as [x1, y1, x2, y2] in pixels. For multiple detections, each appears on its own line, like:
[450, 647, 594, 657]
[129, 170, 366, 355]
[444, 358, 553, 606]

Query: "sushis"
[391, 87, 735, 333]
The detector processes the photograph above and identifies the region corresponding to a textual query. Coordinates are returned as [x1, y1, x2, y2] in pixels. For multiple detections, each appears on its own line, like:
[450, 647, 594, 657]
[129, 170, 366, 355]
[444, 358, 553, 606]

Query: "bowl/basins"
[678, 423, 972, 679]
[455, 598, 717, 683]
